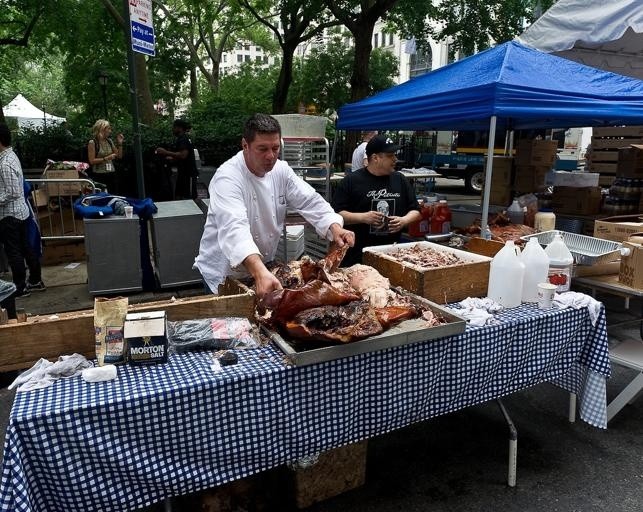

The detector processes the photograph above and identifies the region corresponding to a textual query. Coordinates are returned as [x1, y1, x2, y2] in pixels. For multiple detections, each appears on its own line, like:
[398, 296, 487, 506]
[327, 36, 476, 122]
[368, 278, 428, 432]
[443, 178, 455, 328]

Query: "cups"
[536, 283, 557, 309]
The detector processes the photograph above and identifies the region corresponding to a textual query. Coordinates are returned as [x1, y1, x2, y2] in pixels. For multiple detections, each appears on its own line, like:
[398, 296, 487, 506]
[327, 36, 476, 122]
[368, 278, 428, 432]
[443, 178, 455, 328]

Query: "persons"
[353, 130, 380, 174]
[22, 180, 49, 292]
[88, 120, 124, 195]
[332, 134, 423, 269]
[191, 112, 355, 298]
[1, 122, 47, 298]
[155, 119, 199, 200]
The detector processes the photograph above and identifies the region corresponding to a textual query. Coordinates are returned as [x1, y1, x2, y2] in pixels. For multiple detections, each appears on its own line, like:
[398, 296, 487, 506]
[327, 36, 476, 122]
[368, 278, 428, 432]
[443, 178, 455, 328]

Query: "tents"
[2, 94, 67, 135]
[510, 0, 643, 78]
[336, 41, 643, 239]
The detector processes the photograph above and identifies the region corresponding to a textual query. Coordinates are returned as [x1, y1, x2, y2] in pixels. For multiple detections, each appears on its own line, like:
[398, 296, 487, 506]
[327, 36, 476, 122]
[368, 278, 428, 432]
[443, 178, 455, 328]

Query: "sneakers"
[14, 288, 30, 300]
[26, 281, 46, 292]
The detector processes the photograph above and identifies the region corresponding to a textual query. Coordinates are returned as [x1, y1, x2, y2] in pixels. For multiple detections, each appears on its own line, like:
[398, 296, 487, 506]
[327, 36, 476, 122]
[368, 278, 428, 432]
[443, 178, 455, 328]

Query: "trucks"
[429, 126, 593, 195]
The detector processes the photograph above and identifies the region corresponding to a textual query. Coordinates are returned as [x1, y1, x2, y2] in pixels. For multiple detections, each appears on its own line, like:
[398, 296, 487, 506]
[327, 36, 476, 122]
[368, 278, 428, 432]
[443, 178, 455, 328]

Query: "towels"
[554, 291, 601, 328]
[6, 352, 90, 392]
[451, 296, 502, 328]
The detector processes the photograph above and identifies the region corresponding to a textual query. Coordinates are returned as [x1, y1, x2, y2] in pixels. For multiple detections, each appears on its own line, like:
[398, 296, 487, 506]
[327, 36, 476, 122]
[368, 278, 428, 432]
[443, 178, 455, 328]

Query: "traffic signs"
[124, 0, 157, 200]
[128, 0, 156, 57]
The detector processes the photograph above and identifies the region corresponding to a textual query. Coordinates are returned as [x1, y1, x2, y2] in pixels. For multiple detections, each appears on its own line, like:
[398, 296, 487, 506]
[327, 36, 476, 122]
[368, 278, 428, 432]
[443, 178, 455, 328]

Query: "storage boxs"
[483, 155, 515, 187]
[617, 144, 642, 179]
[515, 139, 559, 166]
[363, 239, 492, 304]
[480, 190, 511, 205]
[553, 186, 602, 215]
[514, 167, 554, 192]
[594, 214, 643, 244]
[46, 169, 81, 197]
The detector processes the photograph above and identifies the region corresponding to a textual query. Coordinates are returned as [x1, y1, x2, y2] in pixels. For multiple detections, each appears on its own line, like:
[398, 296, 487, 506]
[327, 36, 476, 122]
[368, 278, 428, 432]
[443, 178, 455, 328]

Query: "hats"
[365, 134, 403, 156]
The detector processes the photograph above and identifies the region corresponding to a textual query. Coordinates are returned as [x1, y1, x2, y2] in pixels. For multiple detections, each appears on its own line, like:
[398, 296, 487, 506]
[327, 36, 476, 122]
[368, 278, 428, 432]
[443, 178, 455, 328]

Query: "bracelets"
[102, 156, 107, 163]
